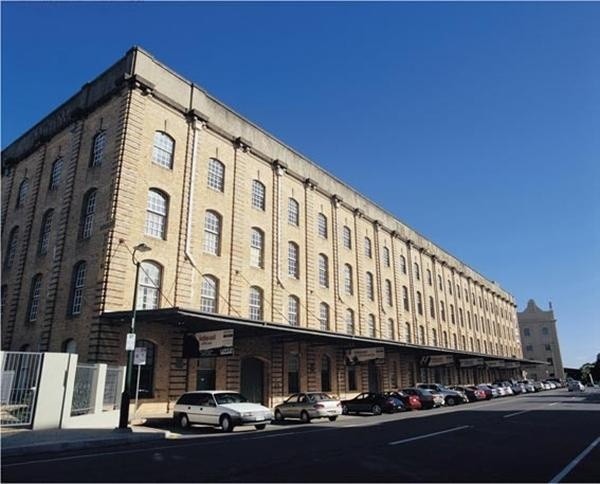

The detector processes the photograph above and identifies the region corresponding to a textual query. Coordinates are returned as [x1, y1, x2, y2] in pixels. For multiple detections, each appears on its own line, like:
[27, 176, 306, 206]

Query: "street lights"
[120, 243, 152, 428]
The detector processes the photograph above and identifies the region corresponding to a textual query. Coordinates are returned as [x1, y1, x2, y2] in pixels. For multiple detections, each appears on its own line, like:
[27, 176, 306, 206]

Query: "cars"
[174, 390, 275, 432]
[275, 391, 343, 422]
[568, 381, 585, 392]
[341, 377, 562, 416]
